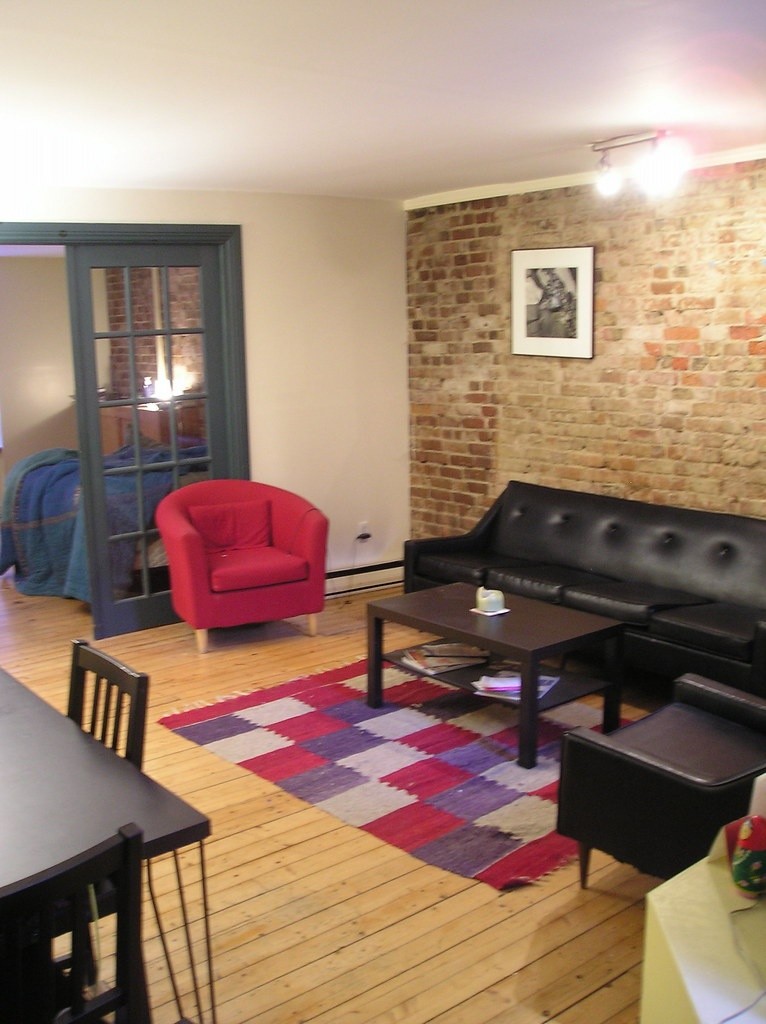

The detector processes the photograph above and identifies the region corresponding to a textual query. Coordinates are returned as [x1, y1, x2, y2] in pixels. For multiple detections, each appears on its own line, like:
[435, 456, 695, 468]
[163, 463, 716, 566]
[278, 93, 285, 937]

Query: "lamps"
[591, 129, 689, 195]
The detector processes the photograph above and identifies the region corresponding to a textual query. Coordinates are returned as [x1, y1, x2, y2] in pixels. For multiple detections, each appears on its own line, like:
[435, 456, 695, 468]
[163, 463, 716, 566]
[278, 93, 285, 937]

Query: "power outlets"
[359, 522, 368, 542]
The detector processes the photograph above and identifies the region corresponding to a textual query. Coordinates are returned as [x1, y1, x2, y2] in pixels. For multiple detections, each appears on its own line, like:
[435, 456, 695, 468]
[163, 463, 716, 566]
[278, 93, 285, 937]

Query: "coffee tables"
[367, 581, 623, 769]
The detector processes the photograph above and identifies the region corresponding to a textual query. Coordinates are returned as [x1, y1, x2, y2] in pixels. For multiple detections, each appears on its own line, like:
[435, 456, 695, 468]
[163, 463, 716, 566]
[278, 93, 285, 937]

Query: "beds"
[0, 434, 212, 602]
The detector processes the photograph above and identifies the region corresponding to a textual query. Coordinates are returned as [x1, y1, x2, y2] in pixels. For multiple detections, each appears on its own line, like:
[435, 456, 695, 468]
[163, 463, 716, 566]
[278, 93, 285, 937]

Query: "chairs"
[154, 479, 329, 650]
[0, 822, 151, 1024]
[557, 673, 766, 889]
[68, 640, 149, 771]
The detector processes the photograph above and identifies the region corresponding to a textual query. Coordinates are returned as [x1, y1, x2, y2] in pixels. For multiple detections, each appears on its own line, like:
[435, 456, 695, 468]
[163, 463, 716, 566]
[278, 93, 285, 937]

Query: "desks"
[156, 658, 636, 890]
[0, 666, 213, 1024]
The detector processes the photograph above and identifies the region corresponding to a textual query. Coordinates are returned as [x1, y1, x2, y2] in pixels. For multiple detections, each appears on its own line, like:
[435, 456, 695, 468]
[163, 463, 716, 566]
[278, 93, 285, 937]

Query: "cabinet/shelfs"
[101, 406, 196, 458]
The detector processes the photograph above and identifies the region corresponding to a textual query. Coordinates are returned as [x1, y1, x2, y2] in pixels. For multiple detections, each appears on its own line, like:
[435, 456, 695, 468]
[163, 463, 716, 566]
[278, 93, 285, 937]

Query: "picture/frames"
[510, 246, 594, 359]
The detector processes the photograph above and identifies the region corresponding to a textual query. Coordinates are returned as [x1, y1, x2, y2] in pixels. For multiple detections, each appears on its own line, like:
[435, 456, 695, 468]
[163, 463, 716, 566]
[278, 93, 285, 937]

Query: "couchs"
[404, 481, 766, 699]
